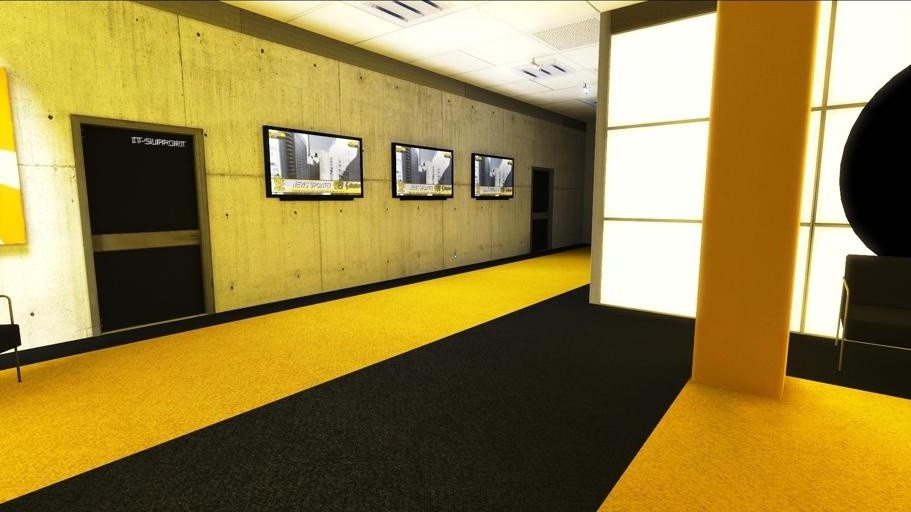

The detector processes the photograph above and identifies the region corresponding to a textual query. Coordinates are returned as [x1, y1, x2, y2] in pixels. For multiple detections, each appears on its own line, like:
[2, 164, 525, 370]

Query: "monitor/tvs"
[263, 125, 363, 201]
[392, 143, 453, 200]
[471, 153, 514, 200]
